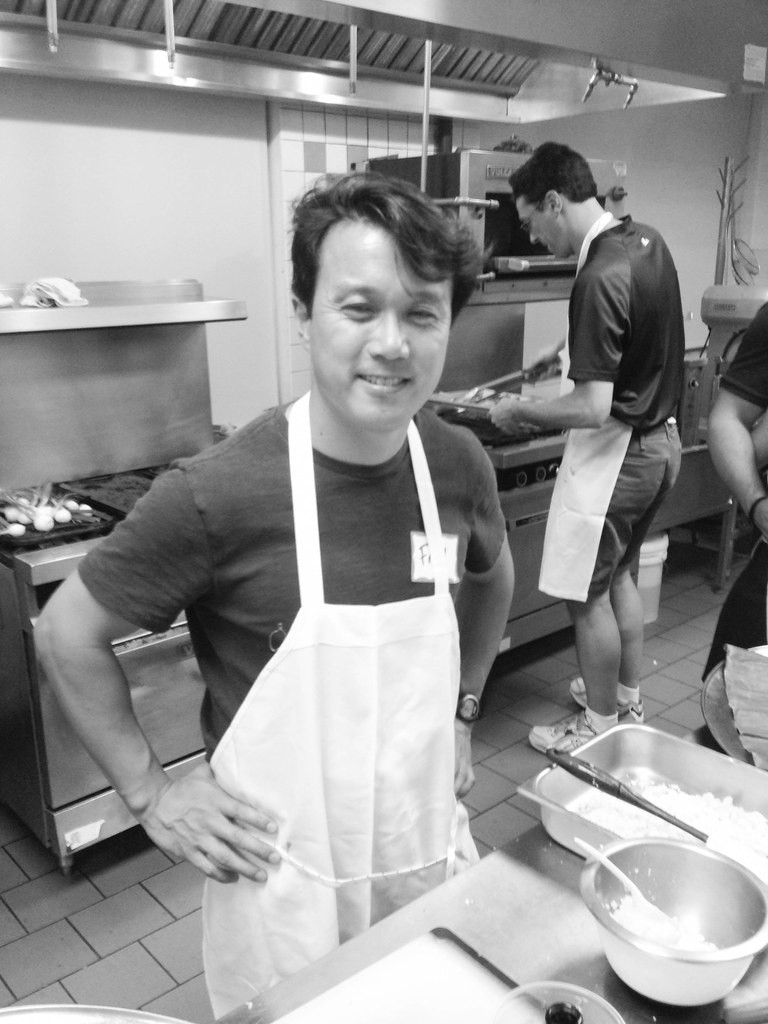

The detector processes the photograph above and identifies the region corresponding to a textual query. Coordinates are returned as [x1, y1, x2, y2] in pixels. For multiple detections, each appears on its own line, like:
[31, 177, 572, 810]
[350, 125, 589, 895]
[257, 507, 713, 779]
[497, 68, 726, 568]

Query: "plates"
[702, 645, 768, 767]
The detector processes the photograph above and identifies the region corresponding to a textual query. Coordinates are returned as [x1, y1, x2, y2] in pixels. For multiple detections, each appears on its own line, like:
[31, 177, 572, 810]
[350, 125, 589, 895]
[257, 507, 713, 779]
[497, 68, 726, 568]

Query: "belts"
[642, 420, 678, 439]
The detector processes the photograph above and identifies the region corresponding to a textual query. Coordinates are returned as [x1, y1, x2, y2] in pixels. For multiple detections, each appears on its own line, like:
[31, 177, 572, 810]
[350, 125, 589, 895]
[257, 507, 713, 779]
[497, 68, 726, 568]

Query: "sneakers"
[527, 710, 597, 756]
[569, 676, 645, 726]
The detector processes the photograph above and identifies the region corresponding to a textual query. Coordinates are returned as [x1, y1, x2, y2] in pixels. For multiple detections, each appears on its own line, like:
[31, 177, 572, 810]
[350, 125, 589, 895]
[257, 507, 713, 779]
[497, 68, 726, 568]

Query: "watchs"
[453, 692, 481, 724]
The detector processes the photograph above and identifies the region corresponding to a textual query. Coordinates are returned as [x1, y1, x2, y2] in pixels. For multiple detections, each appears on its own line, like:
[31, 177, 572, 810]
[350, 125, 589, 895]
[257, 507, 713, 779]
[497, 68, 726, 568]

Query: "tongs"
[458, 358, 562, 413]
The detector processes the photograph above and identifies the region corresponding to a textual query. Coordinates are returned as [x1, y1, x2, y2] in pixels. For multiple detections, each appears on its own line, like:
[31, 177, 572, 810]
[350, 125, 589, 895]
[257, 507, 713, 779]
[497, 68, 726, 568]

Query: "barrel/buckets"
[638, 531, 669, 624]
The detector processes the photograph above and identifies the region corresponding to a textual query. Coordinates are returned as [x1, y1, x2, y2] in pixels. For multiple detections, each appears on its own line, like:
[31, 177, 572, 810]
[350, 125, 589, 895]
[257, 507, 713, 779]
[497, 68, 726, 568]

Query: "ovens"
[370, 146, 628, 305]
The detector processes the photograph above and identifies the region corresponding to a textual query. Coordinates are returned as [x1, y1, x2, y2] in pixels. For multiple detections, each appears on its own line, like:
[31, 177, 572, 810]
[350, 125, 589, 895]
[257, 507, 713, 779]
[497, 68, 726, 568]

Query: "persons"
[25, 169, 515, 1024]
[697, 295, 767, 696]
[491, 138, 687, 757]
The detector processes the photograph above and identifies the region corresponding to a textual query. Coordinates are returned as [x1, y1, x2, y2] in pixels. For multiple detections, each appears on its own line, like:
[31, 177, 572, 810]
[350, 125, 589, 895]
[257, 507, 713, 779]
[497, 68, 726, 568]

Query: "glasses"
[519, 199, 543, 233]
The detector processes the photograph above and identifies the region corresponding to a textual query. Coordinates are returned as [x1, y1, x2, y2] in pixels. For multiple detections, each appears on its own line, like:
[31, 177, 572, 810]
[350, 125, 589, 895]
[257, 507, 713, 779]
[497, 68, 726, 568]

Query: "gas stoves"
[427, 385, 569, 490]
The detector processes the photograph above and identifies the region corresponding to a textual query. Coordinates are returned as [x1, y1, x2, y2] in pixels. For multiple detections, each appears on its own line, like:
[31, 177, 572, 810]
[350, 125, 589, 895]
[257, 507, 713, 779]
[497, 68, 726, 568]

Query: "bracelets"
[746, 493, 767, 522]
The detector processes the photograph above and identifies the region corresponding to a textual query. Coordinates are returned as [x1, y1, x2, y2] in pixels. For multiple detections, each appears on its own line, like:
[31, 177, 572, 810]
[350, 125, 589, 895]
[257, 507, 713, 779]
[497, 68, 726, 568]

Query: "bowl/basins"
[581, 837, 768, 1006]
